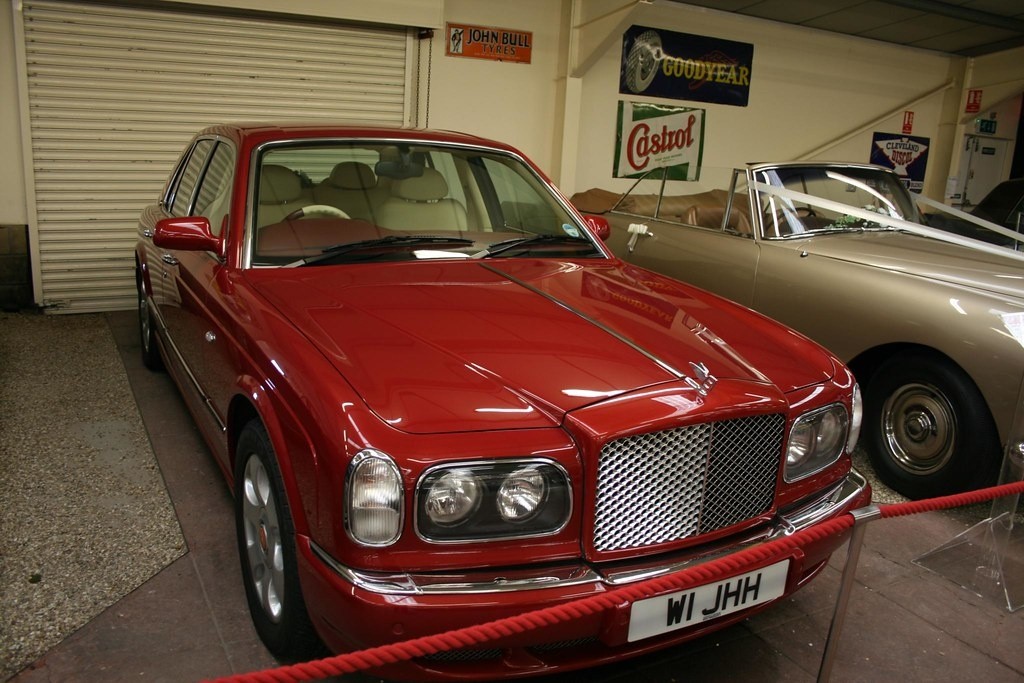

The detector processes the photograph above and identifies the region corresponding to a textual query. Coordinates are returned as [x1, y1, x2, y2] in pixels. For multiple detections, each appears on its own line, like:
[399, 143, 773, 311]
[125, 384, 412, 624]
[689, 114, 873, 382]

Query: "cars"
[496, 160, 1024, 499]
[130, 120, 875, 683]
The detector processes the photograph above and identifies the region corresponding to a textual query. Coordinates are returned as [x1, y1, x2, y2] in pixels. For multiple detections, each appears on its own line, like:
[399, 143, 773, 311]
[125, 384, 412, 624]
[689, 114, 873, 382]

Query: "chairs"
[378, 167, 469, 231]
[682, 205, 752, 235]
[258, 165, 302, 228]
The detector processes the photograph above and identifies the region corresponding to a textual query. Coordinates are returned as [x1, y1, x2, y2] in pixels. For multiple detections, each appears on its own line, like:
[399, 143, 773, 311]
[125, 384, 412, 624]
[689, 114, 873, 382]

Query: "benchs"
[298, 161, 375, 224]
[618, 189, 751, 222]
